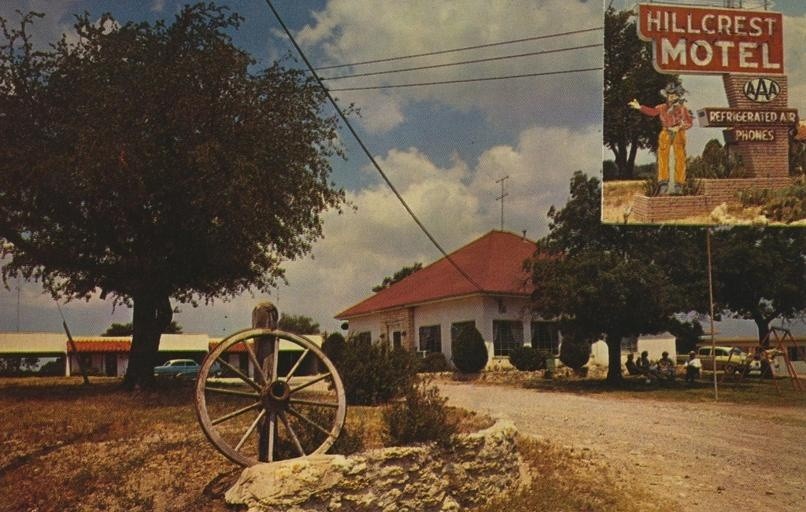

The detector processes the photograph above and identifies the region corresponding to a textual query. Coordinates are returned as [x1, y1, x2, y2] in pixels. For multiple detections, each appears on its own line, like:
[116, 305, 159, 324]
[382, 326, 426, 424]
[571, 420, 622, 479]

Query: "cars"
[154, 359, 201, 379]
[698, 346, 761, 375]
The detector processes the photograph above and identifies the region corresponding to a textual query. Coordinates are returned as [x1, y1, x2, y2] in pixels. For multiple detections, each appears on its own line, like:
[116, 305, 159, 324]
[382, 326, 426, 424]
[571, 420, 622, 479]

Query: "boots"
[659, 182, 681, 196]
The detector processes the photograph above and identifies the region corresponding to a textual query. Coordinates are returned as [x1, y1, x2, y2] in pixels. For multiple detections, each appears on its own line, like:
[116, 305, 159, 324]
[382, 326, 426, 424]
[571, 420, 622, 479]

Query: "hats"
[660, 82, 685, 97]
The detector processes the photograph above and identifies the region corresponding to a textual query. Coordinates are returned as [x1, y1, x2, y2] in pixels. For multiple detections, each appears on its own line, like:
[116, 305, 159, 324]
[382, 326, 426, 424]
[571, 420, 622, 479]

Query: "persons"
[628, 83, 695, 194]
[626, 348, 703, 382]
